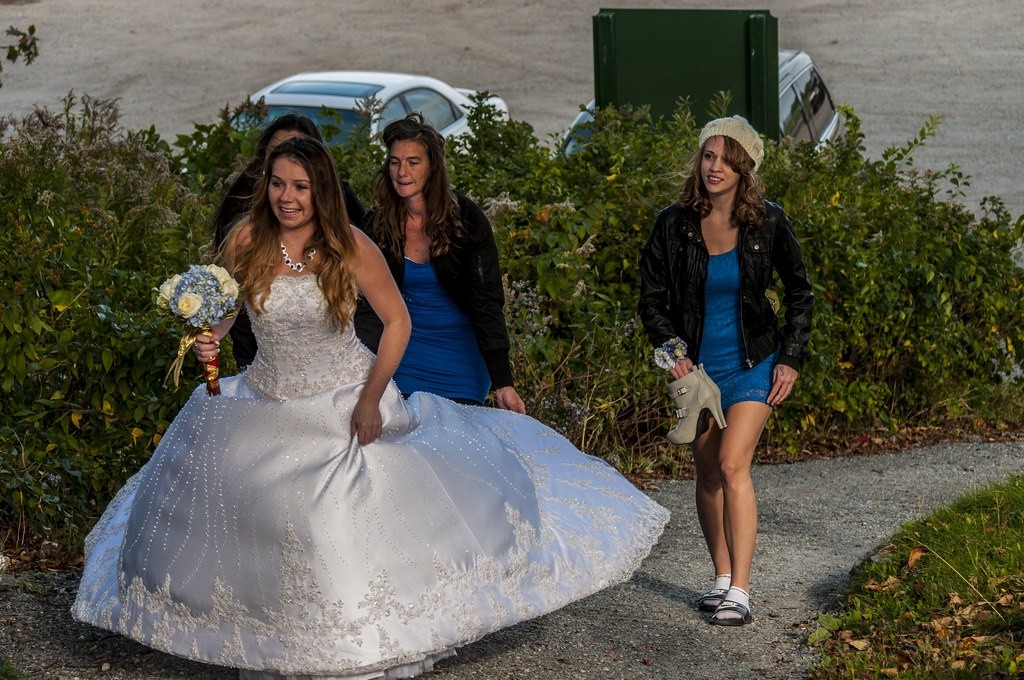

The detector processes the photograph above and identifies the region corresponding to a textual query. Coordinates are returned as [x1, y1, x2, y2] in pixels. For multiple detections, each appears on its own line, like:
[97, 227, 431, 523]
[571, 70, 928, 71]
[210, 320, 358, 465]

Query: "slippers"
[698, 588, 730, 613]
[711, 598, 753, 626]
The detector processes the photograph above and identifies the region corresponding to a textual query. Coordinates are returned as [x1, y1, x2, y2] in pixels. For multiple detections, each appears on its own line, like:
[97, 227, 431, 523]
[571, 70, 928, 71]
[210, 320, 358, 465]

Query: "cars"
[180, 71, 510, 174]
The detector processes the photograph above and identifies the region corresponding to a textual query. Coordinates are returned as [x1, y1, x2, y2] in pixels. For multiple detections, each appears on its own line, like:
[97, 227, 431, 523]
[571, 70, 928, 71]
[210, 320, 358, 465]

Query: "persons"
[70, 134, 671, 680]
[213, 114, 366, 373]
[354, 113, 526, 414]
[635, 115, 814, 625]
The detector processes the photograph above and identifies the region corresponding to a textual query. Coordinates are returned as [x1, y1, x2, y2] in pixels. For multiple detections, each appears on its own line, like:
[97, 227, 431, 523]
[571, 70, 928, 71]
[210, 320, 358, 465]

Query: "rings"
[195, 344, 197, 348]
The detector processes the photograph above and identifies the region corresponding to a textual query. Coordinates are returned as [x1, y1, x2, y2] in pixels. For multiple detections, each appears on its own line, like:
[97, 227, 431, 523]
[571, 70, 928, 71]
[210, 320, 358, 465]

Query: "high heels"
[667, 363, 728, 445]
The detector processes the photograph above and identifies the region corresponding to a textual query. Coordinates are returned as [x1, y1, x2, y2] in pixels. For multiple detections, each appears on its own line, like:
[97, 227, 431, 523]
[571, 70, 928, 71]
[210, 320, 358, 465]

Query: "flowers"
[151, 262, 240, 396]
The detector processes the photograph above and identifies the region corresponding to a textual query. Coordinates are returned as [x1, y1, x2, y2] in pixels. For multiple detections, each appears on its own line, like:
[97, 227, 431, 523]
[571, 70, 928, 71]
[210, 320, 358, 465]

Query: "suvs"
[552, 48, 846, 164]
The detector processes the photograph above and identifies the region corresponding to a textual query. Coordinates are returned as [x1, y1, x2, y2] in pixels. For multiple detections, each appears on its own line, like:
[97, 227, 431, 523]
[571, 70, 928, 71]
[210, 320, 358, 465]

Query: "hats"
[698, 115, 765, 175]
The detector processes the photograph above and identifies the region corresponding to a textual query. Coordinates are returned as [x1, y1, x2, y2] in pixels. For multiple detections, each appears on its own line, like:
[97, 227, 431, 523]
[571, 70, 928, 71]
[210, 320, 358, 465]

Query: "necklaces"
[280, 241, 319, 272]
